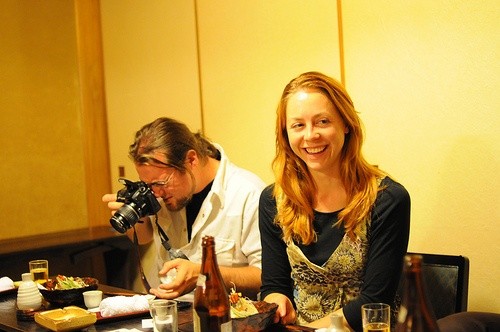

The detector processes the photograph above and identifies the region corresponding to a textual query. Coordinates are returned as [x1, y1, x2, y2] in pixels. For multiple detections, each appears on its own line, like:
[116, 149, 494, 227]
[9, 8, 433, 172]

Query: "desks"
[0, 225, 128, 283]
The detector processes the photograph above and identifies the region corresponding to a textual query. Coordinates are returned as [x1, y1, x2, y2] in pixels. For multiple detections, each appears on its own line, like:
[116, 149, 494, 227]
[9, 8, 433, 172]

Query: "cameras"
[109, 178, 161, 234]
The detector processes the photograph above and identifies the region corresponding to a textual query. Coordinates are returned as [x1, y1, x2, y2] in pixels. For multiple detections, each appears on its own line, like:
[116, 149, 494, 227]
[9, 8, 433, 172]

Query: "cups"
[29, 260, 48, 282]
[361, 303, 390, 332]
[83, 290, 102, 308]
[150, 300, 178, 332]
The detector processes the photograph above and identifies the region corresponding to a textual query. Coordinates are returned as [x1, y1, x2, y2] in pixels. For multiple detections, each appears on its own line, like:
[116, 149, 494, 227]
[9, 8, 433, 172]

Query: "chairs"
[405, 252, 470, 319]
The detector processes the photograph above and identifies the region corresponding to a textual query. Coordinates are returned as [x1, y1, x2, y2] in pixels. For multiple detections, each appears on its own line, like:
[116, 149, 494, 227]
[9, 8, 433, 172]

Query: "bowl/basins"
[39, 278, 98, 308]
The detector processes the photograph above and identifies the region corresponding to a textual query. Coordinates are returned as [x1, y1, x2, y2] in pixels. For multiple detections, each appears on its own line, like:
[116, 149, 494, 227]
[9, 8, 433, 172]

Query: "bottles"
[192, 236, 233, 332]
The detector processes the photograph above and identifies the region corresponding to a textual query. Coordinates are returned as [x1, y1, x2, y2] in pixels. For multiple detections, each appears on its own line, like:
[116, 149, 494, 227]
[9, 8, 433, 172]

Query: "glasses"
[147, 169, 175, 190]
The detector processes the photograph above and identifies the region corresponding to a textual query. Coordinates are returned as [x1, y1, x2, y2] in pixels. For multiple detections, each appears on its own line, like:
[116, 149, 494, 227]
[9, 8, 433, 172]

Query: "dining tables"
[0, 282, 318, 332]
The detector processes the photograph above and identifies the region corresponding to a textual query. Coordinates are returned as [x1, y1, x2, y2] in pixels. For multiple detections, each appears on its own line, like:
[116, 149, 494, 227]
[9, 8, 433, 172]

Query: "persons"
[101, 118, 270, 300]
[258, 71, 412, 332]
